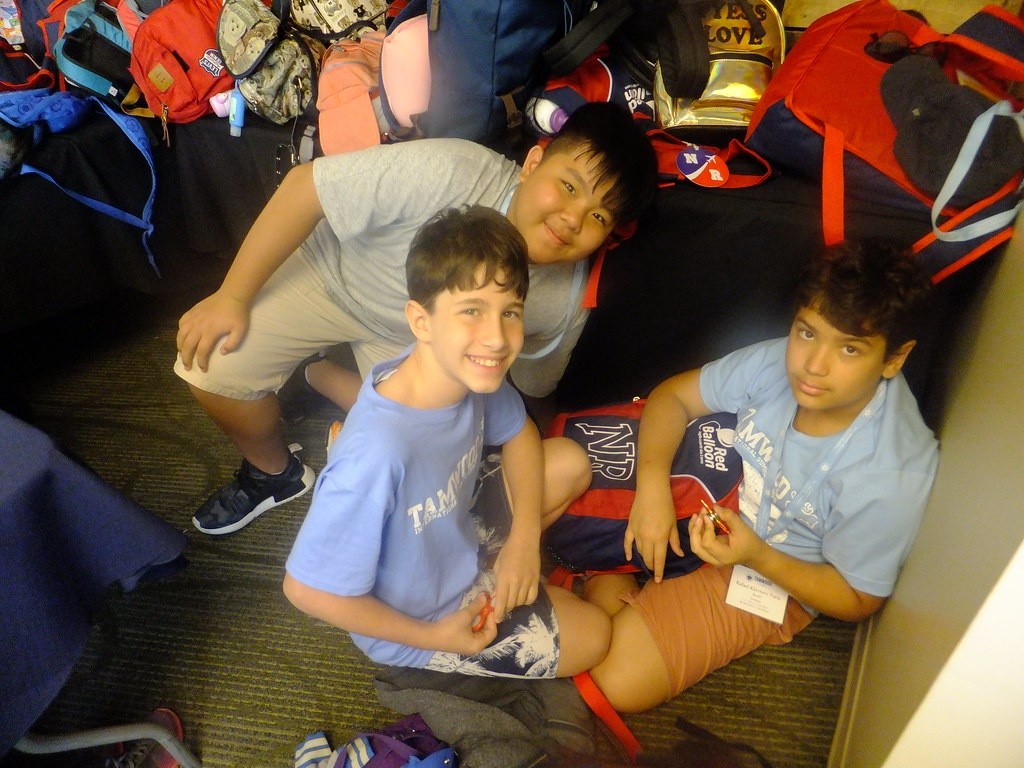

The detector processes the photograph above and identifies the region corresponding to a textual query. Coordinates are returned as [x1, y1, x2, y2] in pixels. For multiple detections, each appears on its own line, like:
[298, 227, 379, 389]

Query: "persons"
[283, 203, 612, 680]
[174, 100, 659, 533]
[541, 232, 939, 713]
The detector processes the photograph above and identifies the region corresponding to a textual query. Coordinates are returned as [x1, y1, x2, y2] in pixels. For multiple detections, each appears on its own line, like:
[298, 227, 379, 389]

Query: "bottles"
[525, 97, 569, 136]
[230, 93, 244, 137]
[209, 88, 239, 118]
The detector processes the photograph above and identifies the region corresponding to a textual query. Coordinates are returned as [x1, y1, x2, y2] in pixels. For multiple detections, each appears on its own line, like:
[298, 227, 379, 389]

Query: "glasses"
[863, 30, 948, 69]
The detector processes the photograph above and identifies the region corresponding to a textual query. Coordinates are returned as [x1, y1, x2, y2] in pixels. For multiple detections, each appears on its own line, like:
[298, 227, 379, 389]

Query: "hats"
[879, 55, 1024, 219]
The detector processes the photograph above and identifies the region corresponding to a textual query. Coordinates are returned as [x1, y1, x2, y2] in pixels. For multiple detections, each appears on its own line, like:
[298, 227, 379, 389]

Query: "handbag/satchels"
[332, 710, 453, 768]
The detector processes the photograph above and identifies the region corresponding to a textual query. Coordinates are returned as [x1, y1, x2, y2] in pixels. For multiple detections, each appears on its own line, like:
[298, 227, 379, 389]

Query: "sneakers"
[275, 347, 332, 426]
[192, 441, 315, 536]
[84, 725, 124, 768]
[104, 709, 184, 768]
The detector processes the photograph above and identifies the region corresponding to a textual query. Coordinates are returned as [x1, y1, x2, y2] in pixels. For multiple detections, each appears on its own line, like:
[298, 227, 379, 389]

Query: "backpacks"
[0, 0, 411, 277]
[536, 397, 745, 581]
[653, 0, 786, 129]
[369, 0, 711, 168]
[745, 0, 1024, 286]
